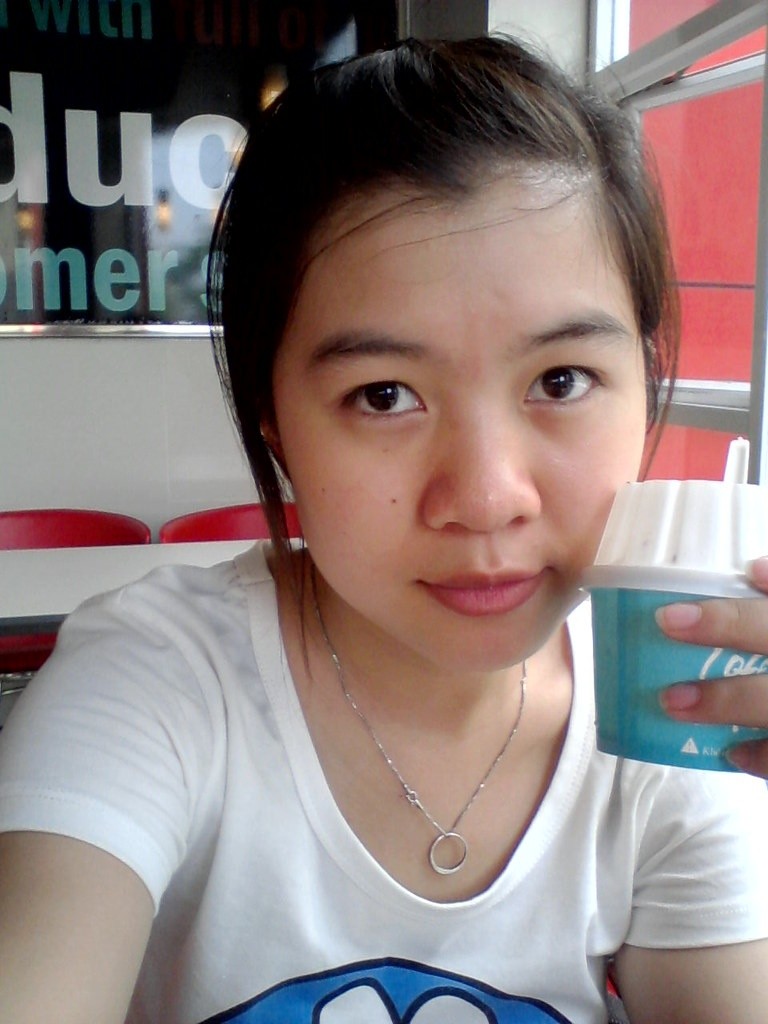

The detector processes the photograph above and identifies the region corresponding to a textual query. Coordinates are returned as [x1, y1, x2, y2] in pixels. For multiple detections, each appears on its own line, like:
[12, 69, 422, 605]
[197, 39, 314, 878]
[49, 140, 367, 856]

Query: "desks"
[0, 535, 306, 633]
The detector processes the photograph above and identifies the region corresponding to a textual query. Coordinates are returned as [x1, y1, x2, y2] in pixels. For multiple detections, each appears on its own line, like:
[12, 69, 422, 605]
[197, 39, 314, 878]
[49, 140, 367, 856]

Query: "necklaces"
[310, 542, 528, 876]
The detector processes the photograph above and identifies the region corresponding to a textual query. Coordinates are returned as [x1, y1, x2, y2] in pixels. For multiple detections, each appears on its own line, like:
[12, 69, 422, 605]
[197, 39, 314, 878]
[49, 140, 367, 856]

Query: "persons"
[2, 38, 767, 1023]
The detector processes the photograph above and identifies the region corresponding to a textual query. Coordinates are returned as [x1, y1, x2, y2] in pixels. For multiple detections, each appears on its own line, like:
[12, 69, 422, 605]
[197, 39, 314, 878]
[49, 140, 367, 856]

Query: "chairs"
[0, 502, 308, 674]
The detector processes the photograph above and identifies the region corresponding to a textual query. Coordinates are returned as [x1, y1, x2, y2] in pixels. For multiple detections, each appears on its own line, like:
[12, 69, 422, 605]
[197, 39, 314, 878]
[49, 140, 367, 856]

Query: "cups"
[579, 480, 768, 772]
